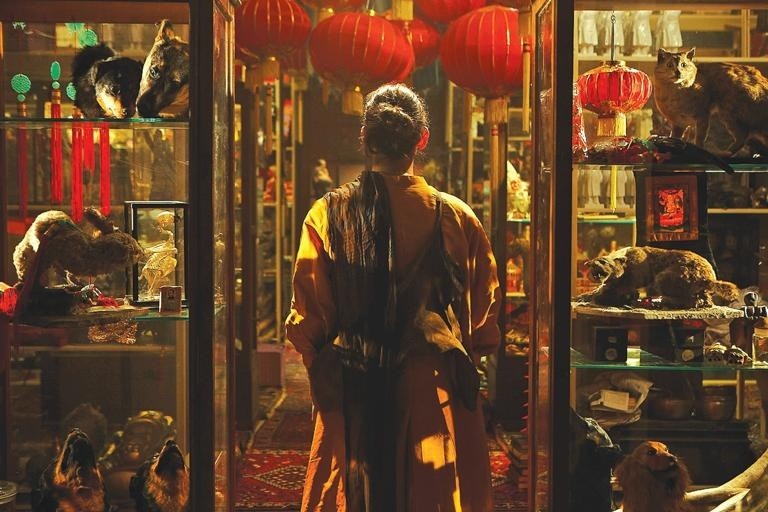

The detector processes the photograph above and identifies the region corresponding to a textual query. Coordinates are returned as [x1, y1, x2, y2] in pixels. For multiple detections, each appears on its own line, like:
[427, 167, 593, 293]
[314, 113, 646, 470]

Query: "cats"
[653, 47, 768, 159]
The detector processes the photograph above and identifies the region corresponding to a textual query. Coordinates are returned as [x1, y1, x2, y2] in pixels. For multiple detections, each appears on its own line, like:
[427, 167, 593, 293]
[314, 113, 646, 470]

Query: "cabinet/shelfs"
[0, 1, 307, 511]
[445, 0, 768, 510]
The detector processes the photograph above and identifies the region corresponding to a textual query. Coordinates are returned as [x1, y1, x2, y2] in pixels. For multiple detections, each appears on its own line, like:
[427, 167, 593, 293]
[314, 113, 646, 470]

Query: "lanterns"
[235, 0, 535, 134]
[577, 60, 653, 138]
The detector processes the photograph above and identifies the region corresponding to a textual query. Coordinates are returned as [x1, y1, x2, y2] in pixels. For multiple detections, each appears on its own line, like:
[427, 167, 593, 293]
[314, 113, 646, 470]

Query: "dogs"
[70, 41, 144, 119]
[30, 428, 114, 512]
[136, 19, 192, 118]
[610, 439, 693, 512]
[127, 439, 190, 512]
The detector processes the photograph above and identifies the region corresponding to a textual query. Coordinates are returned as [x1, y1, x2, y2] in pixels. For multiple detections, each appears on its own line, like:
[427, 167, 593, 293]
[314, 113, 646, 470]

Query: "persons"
[284, 82, 501, 512]
[83, 129, 175, 202]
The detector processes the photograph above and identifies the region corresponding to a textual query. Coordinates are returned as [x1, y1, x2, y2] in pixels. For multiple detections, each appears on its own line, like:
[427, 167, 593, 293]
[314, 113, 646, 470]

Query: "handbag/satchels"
[391, 191, 481, 411]
